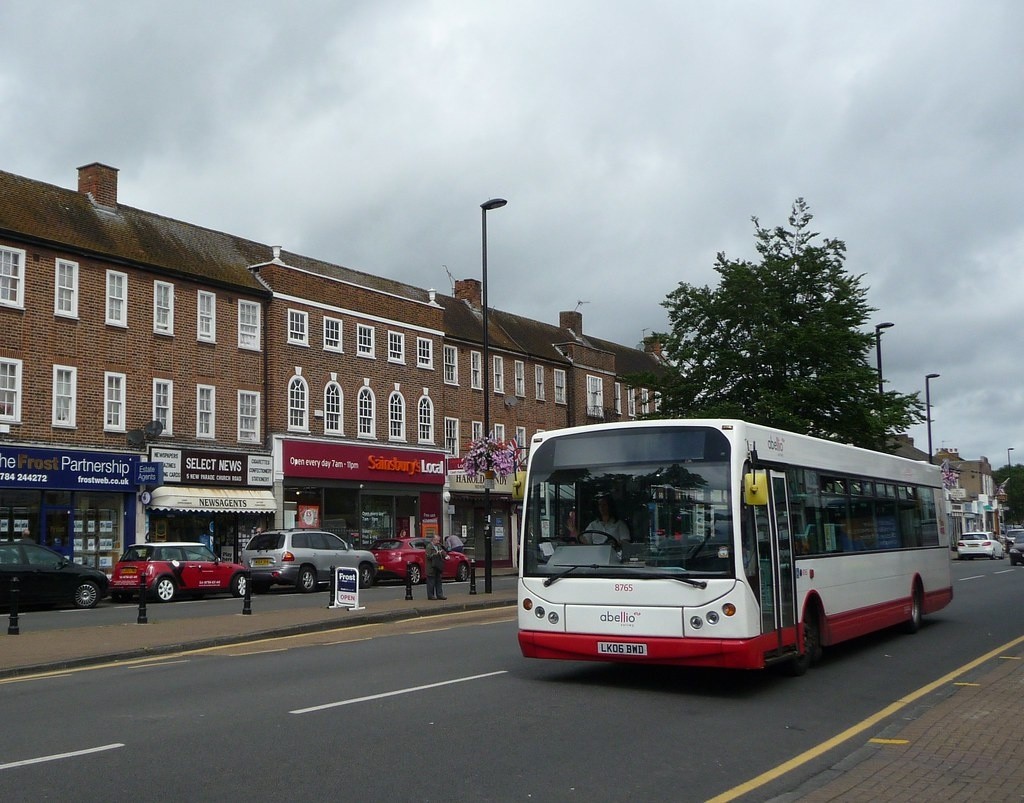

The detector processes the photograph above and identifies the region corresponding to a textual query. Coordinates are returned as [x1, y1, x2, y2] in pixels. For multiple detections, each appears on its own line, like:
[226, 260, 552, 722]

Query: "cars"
[1008, 532, 1024, 566]
[956, 531, 1004, 560]
[0, 539, 111, 609]
[110, 541, 247, 603]
[367, 537, 471, 584]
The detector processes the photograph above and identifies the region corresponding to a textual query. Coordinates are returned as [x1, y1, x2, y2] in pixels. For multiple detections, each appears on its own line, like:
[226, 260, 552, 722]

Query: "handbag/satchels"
[432, 556, 444, 571]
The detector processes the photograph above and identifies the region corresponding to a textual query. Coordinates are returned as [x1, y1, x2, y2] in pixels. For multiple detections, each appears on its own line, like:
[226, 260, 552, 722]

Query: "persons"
[581, 497, 631, 545]
[19, 528, 35, 544]
[400, 530, 406, 538]
[445, 534, 464, 553]
[425, 534, 450, 600]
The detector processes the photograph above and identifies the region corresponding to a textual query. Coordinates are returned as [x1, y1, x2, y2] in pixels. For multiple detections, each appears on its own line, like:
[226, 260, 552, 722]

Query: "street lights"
[924, 373, 941, 464]
[874, 321, 896, 453]
[479, 197, 508, 593]
[1007, 447, 1015, 524]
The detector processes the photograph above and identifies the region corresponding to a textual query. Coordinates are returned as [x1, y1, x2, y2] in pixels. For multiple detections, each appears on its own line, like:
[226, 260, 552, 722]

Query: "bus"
[517, 417, 955, 669]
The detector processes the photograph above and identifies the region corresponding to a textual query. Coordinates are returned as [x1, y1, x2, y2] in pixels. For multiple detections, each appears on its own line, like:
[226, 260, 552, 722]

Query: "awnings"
[145, 484, 277, 513]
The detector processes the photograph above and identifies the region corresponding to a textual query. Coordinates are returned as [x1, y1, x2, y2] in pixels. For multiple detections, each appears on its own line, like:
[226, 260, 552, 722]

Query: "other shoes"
[428, 597, 437, 600]
[437, 596, 447, 600]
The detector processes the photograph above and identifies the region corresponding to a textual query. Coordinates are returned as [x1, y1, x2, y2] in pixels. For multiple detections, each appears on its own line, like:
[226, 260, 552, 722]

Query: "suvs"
[1006, 528, 1024, 554]
[241, 525, 379, 593]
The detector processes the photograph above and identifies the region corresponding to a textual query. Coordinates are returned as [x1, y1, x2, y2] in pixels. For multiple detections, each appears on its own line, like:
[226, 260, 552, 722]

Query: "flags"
[506, 434, 523, 473]
[941, 459, 961, 489]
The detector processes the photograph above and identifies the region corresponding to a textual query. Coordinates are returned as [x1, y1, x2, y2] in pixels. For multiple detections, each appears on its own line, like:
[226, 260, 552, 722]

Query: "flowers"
[459, 436, 521, 485]
